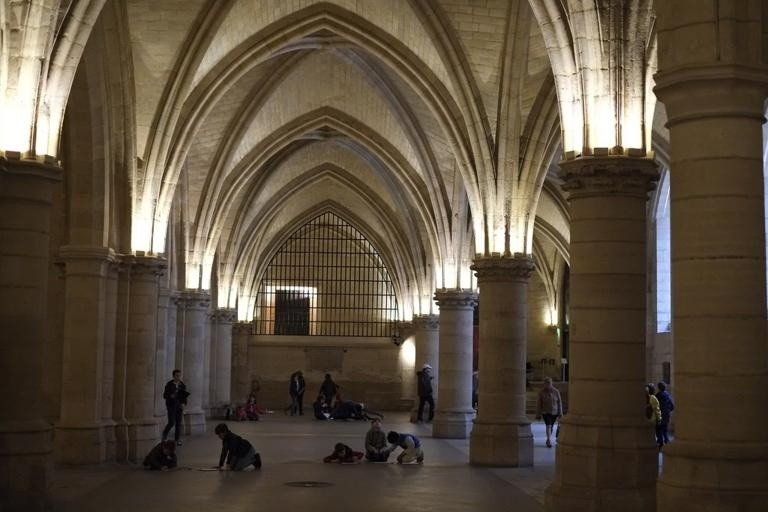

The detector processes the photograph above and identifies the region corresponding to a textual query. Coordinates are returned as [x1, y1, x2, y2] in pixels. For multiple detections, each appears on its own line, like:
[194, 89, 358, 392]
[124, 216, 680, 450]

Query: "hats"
[423, 364, 432, 369]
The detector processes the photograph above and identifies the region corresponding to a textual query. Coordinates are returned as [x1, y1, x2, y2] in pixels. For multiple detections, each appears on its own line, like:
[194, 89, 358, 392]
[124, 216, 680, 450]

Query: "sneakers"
[253, 452, 262, 471]
[416, 451, 424, 465]
[546, 439, 553, 448]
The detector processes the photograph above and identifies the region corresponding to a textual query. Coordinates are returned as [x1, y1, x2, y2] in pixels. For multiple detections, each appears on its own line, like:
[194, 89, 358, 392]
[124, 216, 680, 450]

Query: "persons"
[161, 370, 190, 446]
[236, 393, 265, 421]
[215, 424, 261, 471]
[417, 364, 435, 422]
[284, 371, 305, 416]
[324, 418, 424, 464]
[473, 367, 479, 411]
[646, 382, 674, 448]
[143, 440, 177, 470]
[313, 374, 385, 422]
[535, 377, 562, 448]
